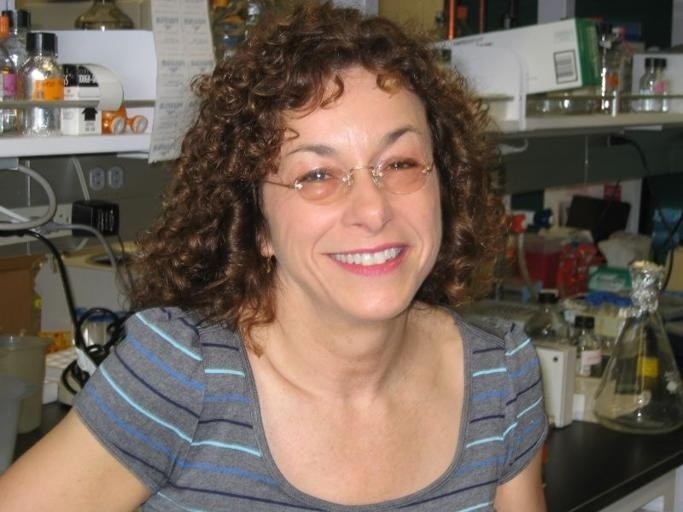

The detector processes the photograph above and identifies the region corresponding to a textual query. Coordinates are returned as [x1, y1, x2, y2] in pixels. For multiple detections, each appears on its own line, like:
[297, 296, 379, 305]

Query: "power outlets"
[37, 200, 75, 241]
[88, 166, 107, 193]
[605, 130, 623, 147]
[106, 166, 127, 191]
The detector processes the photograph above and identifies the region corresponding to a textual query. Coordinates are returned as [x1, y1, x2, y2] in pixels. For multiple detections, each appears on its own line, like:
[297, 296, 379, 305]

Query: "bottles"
[599, 27, 633, 116]
[0, 8, 65, 139]
[569, 316, 601, 377]
[637, 57, 668, 114]
[523, 292, 568, 342]
[590, 262, 683, 434]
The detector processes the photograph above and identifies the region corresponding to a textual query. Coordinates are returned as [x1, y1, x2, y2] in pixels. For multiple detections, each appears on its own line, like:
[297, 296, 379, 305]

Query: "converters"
[74, 202, 119, 236]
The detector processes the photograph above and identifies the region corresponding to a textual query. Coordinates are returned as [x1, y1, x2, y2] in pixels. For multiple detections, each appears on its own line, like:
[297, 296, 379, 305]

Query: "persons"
[0, 5, 553, 511]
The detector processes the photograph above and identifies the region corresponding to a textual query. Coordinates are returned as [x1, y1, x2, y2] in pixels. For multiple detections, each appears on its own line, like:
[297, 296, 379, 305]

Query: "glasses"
[261, 160, 437, 206]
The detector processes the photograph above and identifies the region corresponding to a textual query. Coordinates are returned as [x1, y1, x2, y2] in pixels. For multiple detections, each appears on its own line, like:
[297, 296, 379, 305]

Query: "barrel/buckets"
[0, 334, 55, 478]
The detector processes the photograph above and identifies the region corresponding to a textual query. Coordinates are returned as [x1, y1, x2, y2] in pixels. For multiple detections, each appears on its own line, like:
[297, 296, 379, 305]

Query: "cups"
[0, 333, 50, 432]
[0, 375, 26, 475]
